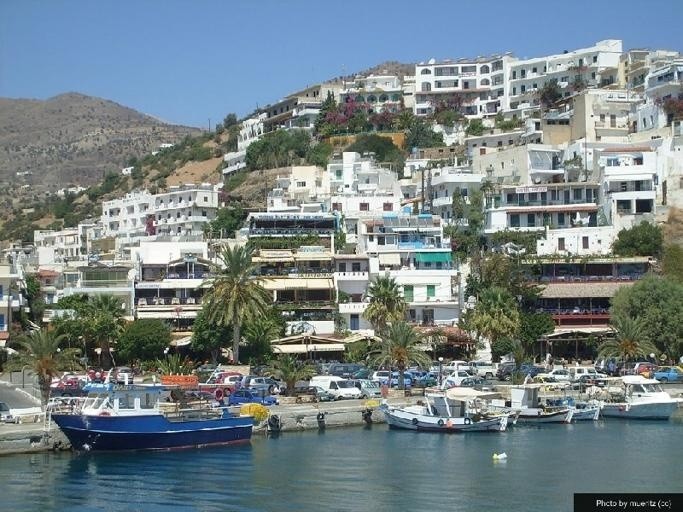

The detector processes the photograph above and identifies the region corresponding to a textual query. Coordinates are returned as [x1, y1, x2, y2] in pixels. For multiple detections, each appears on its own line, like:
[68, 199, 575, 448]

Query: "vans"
[0, 402, 14, 423]
[245, 377, 279, 395]
[109, 367, 135, 384]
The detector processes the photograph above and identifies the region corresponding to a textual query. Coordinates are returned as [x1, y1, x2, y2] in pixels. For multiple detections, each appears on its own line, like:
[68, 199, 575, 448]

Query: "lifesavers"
[215, 388, 224, 400]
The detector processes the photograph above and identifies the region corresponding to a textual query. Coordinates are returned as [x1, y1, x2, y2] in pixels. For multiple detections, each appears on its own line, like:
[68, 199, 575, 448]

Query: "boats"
[538, 397, 603, 422]
[488, 382, 575, 423]
[380, 385, 512, 432]
[46, 376, 256, 451]
[574, 374, 683, 418]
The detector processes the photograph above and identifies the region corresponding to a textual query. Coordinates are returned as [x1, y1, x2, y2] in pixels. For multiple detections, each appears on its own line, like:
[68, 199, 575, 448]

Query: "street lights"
[438, 356, 444, 385]
[649, 353, 655, 373]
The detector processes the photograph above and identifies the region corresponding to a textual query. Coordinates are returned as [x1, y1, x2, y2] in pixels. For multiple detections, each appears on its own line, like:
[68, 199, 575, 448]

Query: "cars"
[215, 361, 439, 404]
[445, 360, 683, 387]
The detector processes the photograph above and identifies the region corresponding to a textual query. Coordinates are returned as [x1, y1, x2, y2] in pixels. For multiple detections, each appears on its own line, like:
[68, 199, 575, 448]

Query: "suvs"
[88, 366, 106, 384]
[50, 371, 88, 397]
